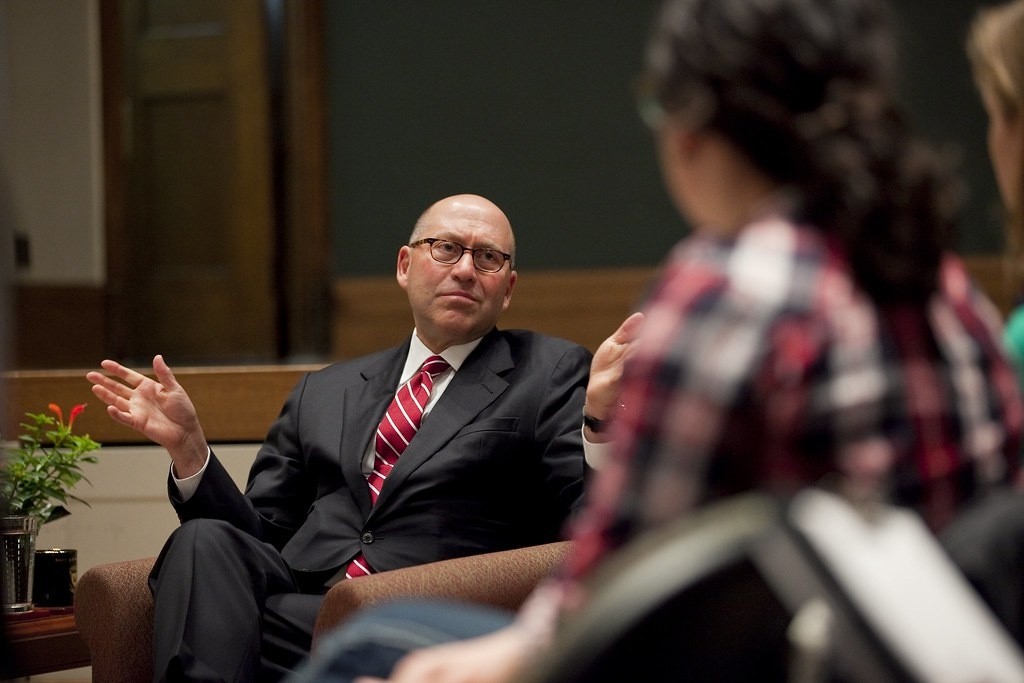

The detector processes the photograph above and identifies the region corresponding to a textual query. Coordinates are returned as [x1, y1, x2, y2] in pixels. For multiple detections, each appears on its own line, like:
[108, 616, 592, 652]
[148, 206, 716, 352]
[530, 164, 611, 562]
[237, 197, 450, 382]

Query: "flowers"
[0, 400, 103, 520]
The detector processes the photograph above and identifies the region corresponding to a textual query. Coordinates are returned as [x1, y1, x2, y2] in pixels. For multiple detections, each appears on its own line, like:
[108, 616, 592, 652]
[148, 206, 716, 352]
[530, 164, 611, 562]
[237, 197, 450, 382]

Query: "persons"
[282, 0, 1024, 683]
[88, 194, 658, 683]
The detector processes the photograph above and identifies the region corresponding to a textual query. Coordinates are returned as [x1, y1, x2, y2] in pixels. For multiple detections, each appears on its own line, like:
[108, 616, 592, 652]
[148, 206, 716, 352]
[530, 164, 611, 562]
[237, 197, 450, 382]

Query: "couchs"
[74, 540, 575, 683]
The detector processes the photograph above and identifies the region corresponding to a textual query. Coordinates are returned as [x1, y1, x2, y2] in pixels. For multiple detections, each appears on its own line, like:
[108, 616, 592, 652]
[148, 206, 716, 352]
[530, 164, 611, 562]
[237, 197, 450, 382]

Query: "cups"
[32, 548, 77, 610]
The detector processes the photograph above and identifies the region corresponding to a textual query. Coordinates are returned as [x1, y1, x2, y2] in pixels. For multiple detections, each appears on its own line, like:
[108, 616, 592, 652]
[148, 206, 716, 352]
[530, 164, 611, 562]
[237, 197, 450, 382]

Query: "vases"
[0, 515, 41, 612]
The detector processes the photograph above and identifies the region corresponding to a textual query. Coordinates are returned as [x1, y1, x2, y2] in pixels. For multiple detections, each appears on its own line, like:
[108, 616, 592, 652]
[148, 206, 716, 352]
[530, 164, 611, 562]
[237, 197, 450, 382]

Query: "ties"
[345, 355, 451, 580]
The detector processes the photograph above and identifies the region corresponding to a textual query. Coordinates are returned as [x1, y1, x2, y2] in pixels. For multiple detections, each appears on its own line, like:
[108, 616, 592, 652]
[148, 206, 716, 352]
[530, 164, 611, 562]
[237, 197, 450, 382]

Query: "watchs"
[582, 403, 627, 435]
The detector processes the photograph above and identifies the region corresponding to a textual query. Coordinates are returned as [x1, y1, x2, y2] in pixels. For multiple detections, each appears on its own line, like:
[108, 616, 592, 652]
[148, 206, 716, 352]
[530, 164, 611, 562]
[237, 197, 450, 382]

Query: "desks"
[0, 606, 93, 683]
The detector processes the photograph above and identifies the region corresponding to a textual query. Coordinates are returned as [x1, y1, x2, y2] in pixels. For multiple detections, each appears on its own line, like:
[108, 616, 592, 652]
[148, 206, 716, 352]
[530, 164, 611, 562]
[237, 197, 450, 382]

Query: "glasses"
[408, 238, 513, 273]
